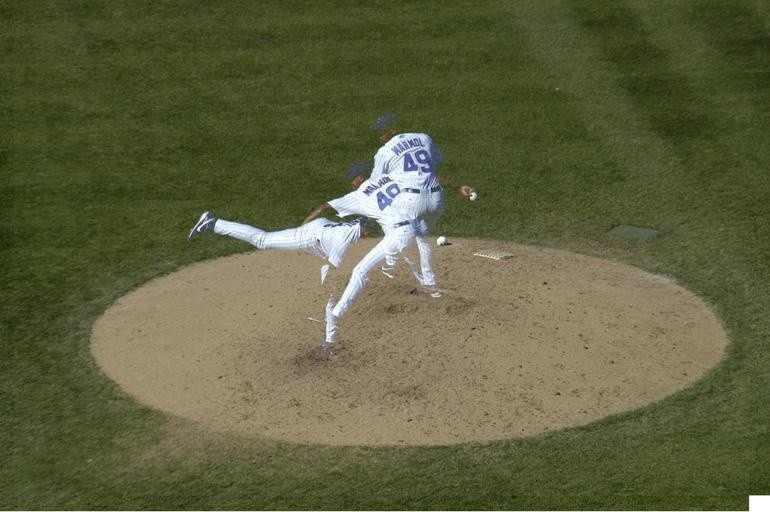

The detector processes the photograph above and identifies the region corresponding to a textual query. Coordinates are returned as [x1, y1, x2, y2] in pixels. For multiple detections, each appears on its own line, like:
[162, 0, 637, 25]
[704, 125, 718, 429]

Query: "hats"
[342, 160, 373, 180]
[368, 112, 401, 136]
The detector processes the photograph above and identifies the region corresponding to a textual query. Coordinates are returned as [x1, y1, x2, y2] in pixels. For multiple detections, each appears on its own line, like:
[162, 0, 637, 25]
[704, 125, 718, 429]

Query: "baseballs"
[439, 236, 446, 245]
[469, 192, 476, 200]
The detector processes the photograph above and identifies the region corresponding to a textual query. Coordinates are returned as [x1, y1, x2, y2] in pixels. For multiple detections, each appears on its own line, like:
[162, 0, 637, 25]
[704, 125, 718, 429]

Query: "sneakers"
[185, 210, 217, 241]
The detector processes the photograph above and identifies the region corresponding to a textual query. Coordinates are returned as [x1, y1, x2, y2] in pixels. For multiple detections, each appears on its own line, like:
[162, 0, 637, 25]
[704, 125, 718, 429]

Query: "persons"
[301, 158, 475, 360]
[365, 111, 450, 294]
[184, 206, 382, 323]
